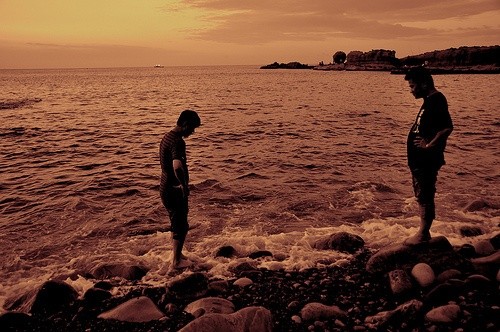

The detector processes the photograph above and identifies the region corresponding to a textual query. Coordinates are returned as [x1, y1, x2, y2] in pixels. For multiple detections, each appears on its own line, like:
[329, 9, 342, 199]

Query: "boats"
[391, 68, 410, 74]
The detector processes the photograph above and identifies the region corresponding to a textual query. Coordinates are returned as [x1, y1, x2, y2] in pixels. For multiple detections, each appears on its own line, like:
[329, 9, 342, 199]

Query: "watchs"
[426, 144, 429, 147]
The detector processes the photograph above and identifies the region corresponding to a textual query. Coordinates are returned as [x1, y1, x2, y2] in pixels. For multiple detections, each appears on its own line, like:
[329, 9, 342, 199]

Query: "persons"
[403, 67, 453, 245]
[159, 110, 201, 268]
